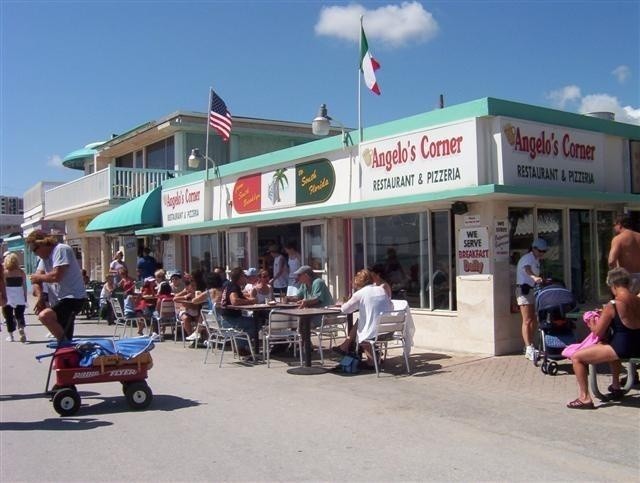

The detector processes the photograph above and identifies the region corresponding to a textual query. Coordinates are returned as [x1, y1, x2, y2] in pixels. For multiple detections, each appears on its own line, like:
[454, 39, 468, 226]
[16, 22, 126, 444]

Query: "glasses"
[536, 247, 547, 253]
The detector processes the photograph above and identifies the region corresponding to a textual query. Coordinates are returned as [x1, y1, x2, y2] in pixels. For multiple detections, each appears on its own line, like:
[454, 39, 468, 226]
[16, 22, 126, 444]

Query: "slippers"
[567, 399, 595, 409]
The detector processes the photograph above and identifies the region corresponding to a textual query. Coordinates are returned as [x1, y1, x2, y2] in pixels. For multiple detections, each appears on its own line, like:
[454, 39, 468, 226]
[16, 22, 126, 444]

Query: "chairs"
[200, 299, 411, 377]
[80, 281, 203, 342]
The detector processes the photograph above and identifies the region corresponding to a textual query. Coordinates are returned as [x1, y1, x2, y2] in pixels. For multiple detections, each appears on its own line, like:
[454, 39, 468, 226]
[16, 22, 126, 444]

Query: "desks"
[275, 307, 341, 374]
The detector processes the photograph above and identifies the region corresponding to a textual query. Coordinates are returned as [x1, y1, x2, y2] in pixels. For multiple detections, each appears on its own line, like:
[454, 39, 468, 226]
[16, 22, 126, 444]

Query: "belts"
[515, 283, 534, 290]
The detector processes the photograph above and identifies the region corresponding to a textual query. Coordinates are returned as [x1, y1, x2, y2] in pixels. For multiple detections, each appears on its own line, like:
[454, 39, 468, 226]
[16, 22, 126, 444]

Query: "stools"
[587, 355, 638, 402]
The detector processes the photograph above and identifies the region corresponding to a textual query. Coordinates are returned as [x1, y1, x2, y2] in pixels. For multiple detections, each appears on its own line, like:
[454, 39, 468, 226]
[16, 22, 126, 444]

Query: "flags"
[208, 88, 233, 143]
[358, 23, 383, 96]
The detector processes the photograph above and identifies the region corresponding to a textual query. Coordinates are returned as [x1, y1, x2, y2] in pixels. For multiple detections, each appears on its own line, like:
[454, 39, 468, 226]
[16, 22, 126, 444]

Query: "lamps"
[310, 99, 357, 154]
[186, 145, 223, 185]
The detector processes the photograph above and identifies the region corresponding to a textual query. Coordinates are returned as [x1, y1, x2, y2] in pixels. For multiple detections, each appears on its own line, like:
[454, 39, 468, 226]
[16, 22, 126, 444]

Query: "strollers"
[533, 276, 580, 375]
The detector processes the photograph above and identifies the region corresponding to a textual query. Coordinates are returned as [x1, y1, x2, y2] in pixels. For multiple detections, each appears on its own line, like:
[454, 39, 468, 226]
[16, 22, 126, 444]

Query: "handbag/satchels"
[339, 355, 359, 373]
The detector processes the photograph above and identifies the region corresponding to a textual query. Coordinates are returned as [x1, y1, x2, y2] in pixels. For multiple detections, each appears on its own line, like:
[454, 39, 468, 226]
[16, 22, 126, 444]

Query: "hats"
[293, 265, 312, 275]
[244, 268, 257, 276]
[533, 238, 549, 251]
[265, 244, 279, 253]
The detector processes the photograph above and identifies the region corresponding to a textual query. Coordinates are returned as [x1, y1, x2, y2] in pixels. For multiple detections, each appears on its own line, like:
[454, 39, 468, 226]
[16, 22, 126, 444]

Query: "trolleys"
[45, 300, 154, 416]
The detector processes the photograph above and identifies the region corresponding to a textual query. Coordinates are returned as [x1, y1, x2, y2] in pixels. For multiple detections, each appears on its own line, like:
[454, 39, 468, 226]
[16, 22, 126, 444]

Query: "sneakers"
[525, 345, 540, 361]
[185, 331, 202, 340]
[359, 361, 375, 369]
[19, 329, 26, 342]
[332, 346, 346, 354]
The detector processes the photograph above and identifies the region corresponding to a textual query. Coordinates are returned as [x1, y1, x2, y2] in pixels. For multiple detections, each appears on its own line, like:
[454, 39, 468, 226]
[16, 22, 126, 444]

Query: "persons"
[287, 264, 334, 352]
[376, 248, 445, 306]
[82, 270, 89, 314]
[4, 252, 28, 342]
[513, 236, 551, 362]
[0, 259, 8, 306]
[267, 241, 302, 297]
[332, 263, 391, 370]
[565, 266, 639, 408]
[33, 257, 58, 338]
[100, 268, 275, 356]
[137, 248, 157, 281]
[108, 252, 128, 286]
[606, 209, 640, 387]
[26, 228, 89, 346]
